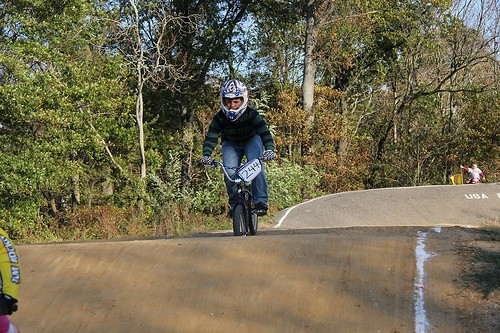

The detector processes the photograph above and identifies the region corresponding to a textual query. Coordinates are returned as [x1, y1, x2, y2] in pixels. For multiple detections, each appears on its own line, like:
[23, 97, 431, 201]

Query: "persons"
[0, 225, 24, 332]
[201, 78, 277, 220]
[461, 162, 488, 186]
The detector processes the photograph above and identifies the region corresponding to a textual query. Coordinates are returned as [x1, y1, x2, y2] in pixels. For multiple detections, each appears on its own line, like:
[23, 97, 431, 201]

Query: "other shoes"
[228, 209, 233, 218]
[256, 207, 267, 216]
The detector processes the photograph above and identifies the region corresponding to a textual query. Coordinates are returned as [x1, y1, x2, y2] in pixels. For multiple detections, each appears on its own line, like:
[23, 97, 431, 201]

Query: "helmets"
[220, 79, 248, 122]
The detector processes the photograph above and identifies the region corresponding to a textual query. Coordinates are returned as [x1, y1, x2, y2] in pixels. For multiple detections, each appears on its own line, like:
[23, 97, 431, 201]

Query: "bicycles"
[202, 155, 279, 237]
[466, 178, 485, 184]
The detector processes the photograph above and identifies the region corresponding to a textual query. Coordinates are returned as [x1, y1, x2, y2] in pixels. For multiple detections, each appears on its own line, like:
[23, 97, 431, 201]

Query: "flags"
[449, 174, 465, 185]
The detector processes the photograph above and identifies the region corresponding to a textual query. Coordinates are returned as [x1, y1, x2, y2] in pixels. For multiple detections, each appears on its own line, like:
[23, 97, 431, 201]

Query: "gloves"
[201, 156, 212, 165]
[263, 150, 274, 161]
